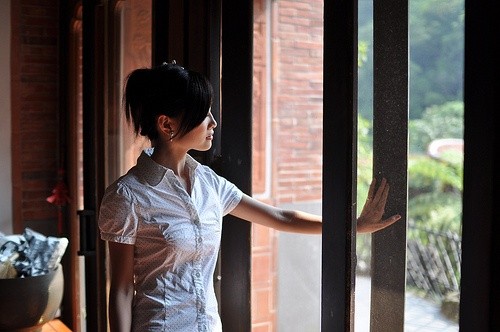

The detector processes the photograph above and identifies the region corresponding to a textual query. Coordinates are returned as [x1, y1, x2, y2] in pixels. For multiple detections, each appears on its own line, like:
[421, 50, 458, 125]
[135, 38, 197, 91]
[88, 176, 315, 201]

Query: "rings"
[366, 198, 373, 202]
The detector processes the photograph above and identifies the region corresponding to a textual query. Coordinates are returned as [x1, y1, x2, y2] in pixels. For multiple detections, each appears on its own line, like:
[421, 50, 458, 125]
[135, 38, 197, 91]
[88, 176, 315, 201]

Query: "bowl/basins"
[0, 263, 64, 325]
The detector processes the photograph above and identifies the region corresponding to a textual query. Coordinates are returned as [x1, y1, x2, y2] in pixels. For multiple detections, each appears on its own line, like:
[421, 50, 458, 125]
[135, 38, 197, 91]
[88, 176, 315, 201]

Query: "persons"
[98, 61, 402, 332]
